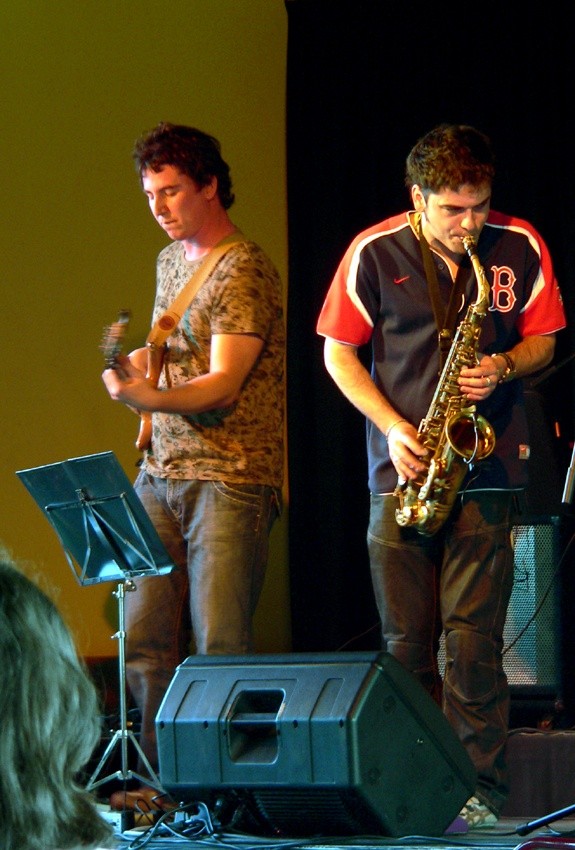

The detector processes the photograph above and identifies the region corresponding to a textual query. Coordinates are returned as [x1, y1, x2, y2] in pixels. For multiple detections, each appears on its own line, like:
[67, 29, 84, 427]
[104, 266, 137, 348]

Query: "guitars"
[97, 309, 167, 452]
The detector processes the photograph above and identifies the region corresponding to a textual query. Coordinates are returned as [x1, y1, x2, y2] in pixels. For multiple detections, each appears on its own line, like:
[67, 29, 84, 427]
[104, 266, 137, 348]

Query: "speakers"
[435, 512, 575, 715]
[154, 651, 478, 841]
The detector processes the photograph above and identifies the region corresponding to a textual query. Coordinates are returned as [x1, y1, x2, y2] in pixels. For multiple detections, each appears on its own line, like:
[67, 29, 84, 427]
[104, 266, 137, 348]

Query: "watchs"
[491, 353, 516, 384]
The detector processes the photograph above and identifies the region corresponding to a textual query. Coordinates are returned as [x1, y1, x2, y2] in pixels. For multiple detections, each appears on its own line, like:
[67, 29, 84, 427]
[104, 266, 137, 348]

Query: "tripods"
[43, 489, 177, 810]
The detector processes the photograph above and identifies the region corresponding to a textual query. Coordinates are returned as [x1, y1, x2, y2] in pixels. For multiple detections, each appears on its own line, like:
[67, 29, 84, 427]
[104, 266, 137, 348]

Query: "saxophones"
[392, 235, 496, 538]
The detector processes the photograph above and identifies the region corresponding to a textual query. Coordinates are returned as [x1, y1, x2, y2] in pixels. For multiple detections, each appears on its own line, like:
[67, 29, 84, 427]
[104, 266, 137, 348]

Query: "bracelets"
[385, 420, 406, 437]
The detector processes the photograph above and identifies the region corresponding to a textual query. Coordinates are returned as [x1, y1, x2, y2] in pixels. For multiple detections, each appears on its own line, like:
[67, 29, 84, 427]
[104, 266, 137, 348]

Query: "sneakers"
[459, 796, 497, 829]
[110, 786, 179, 815]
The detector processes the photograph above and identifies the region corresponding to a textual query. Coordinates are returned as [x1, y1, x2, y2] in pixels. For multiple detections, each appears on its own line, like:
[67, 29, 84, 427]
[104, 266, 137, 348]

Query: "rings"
[487, 378, 490, 386]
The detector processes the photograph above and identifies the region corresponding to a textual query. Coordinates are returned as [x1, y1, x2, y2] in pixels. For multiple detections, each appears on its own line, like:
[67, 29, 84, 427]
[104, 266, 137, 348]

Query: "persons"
[103, 125, 286, 820]
[317, 122, 568, 826]
[0, 562, 115, 850]
